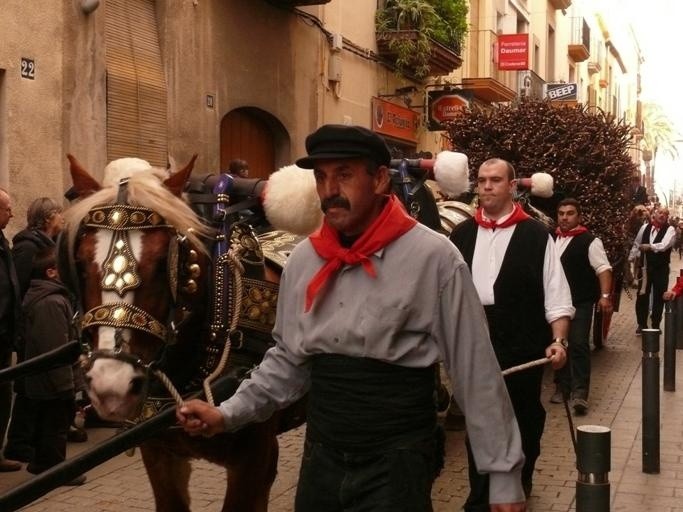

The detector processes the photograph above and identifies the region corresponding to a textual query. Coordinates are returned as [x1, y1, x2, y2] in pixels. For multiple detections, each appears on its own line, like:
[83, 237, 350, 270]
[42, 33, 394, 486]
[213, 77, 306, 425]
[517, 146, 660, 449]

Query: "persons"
[547, 198, 614, 411]
[624, 204, 683, 335]
[231, 159, 249, 178]
[0, 186, 102, 486]
[446, 158, 578, 511]
[173, 124, 529, 510]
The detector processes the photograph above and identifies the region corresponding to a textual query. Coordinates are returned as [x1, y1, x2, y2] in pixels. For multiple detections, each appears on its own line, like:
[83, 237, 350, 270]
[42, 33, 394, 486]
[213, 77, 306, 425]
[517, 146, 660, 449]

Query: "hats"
[296, 124, 391, 171]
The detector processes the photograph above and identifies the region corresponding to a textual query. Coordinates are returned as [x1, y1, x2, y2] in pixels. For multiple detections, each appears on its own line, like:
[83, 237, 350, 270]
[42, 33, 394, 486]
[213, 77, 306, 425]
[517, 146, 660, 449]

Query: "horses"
[55, 149, 307, 512]
[627, 202, 652, 286]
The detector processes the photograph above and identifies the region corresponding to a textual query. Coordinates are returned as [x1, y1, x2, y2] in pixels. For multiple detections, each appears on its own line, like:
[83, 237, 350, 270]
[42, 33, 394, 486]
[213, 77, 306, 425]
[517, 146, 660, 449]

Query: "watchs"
[601, 293, 613, 299]
[550, 337, 569, 351]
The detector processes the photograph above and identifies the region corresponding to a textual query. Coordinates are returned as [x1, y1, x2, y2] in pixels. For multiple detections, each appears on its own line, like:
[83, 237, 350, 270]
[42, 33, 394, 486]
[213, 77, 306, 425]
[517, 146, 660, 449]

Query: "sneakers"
[551, 382, 589, 412]
[636, 326, 648, 336]
[0, 426, 87, 485]
